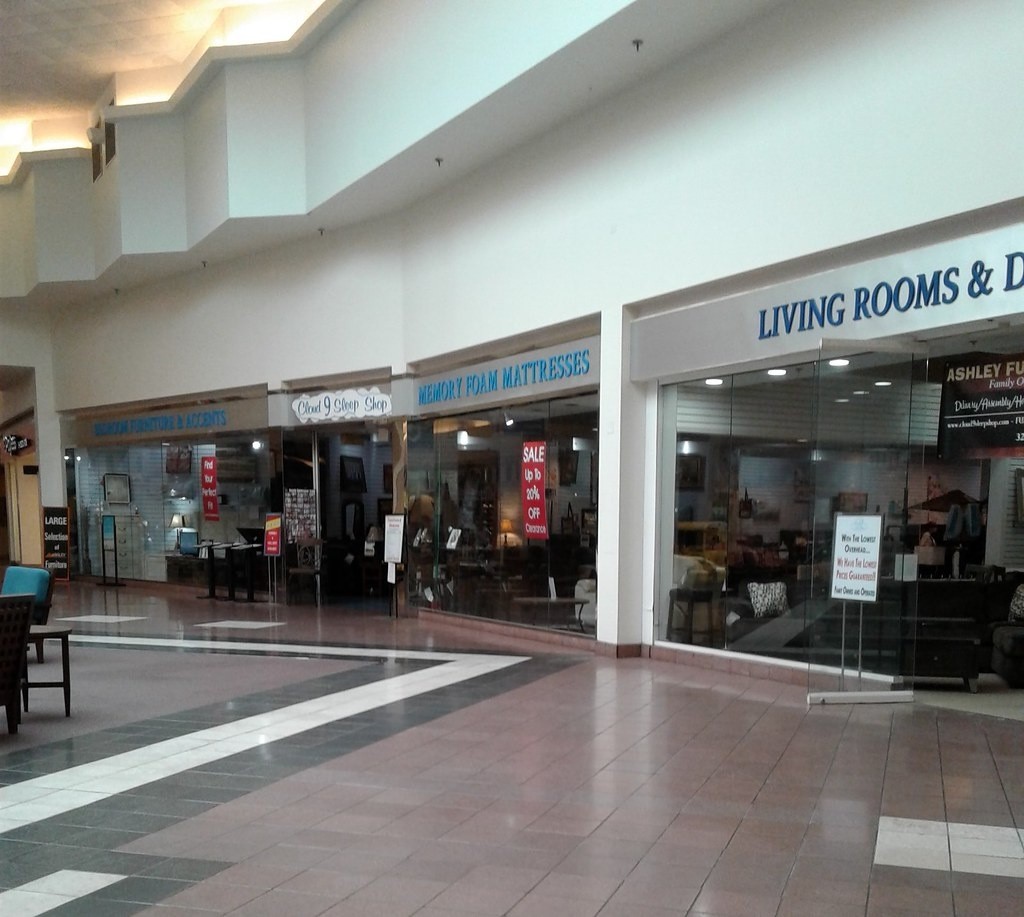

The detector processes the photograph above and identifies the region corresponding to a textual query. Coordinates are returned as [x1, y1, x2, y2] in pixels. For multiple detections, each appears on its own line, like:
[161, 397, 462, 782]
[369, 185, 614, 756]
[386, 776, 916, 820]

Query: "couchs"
[723, 577, 984, 693]
[575, 579, 596, 628]
[985, 583, 1024, 688]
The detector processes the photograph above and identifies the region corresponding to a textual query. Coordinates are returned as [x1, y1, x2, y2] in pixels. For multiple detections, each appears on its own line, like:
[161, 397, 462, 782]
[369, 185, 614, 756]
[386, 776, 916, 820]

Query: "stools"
[670, 589, 715, 648]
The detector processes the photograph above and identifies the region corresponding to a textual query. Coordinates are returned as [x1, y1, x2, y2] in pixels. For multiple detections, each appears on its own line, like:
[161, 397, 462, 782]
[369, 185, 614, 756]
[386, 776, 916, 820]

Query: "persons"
[926, 475, 944, 501]
[919, 521, 936, 579]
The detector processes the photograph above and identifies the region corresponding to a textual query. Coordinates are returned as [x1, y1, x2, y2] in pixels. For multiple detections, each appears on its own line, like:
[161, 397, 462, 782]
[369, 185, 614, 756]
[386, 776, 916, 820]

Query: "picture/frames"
[340, 455, 368, 494]
[382, 463, 406, 492]
[677, 454, 706, 493]
[837, 491, 869, 513]
[103, 473, 132, 504]
[377, 497, 393, 527]
[560, 447, 580, 485]
[1016, 468, 1024, 523]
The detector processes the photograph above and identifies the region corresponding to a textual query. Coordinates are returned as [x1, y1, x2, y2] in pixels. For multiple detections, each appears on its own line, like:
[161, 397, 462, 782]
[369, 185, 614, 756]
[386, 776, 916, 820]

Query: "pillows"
[1007, 583, 1024, 624]
[747, 580, 791, 618]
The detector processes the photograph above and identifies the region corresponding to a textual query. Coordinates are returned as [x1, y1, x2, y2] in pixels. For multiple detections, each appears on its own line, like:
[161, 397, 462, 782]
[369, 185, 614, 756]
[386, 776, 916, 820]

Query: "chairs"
[287, 539, 324, 605]
[0, 592, 37, 736]
[0, 565, 57, 664]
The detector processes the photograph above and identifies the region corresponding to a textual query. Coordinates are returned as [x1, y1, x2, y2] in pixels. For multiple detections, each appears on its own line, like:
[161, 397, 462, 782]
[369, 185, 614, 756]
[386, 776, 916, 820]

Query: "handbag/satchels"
[739, 489, 757, 519]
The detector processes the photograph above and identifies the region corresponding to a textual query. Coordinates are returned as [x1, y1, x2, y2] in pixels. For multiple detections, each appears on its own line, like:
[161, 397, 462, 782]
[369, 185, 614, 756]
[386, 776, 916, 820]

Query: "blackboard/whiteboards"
[937, 354, 1024, 458]
[828, 511, 886, 604]
[39, 505, 72, 581]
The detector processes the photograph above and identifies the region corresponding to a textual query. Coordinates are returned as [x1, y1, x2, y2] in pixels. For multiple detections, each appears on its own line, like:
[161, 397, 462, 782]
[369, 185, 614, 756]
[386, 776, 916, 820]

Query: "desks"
[25, 625, 73, 717]
[513, 595, 590, 634]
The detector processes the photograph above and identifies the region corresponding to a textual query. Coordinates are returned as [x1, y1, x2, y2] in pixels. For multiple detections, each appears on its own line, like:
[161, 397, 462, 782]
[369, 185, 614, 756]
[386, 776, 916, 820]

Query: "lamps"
[366, 526, 384, 543]
[170, 513, 186, 551]
[500, 520, 513, 548]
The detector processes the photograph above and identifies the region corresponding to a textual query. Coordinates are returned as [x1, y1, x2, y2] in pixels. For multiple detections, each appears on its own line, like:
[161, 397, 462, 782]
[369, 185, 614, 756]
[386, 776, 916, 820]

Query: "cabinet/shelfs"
[458, 449, 500, 546]
